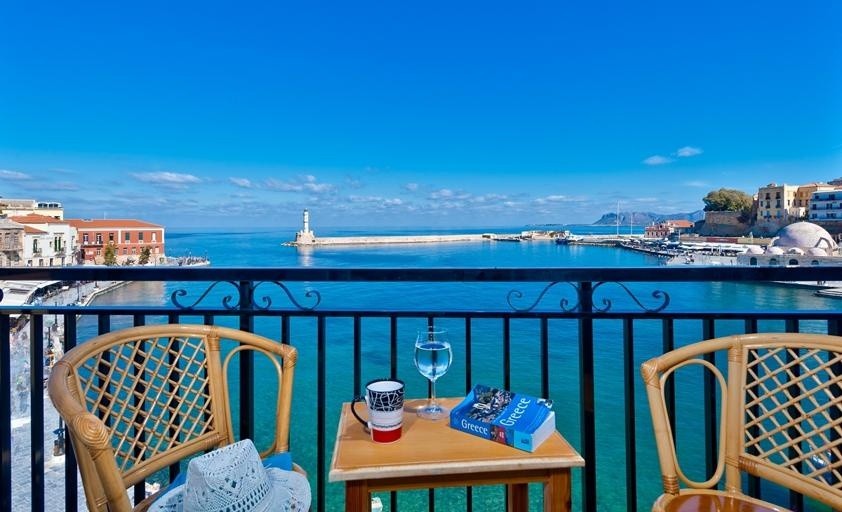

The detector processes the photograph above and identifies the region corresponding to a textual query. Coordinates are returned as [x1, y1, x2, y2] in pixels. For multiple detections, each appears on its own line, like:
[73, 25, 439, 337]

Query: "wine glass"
[412, 325, 455, 424]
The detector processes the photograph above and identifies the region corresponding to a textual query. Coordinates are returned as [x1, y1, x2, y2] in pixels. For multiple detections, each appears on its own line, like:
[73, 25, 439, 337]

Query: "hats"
[145, 438, 312, 512]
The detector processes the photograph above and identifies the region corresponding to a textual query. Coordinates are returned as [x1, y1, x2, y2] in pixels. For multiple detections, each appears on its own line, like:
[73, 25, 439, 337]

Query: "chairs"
[48, 324, 307, 512]
[640, 332, 842, 512]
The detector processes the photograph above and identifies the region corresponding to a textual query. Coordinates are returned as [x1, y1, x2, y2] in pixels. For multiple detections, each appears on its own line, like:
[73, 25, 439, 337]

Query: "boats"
[555, 235, 583, 244]
[491, 236, 523, 242]
[808, 445, 832, 486]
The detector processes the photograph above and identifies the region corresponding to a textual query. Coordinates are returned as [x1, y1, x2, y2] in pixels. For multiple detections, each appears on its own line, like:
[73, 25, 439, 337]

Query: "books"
[450, 384, 556, 455]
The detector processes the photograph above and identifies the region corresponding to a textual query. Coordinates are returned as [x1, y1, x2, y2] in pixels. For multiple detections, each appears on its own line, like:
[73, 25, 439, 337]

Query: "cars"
[27, 367, 51, 390]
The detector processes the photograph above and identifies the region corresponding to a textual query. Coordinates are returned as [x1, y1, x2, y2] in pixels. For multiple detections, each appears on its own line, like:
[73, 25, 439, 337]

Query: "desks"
[328, 397, 586, 512]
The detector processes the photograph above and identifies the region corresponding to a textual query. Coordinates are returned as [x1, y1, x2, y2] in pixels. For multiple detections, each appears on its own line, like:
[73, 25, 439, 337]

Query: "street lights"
[52, 296, 60, 331]
[44, 320, 53, 361]
[75, 280, 80, 303]
[189, 251, 192, 264]
[185, 248, 189, 258]
[205, 249, 207, 261]
[168, 247, 173, 256]
[154, 254, 157, 266]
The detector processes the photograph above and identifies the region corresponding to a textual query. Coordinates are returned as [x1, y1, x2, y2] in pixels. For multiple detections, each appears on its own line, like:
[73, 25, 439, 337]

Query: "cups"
[348, 376, 410, 448]
